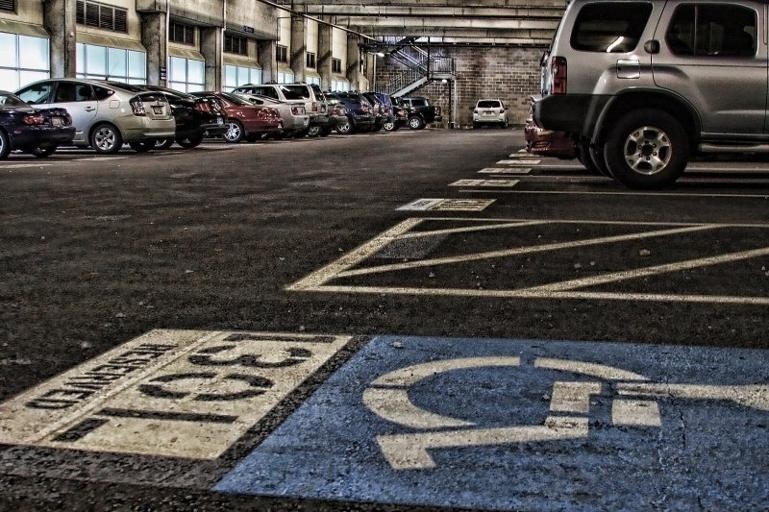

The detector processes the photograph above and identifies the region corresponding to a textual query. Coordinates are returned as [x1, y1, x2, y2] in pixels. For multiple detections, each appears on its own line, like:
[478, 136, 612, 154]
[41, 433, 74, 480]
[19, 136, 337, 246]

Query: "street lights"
[442, 78, 451, 130]
[373, 52, 384, 91]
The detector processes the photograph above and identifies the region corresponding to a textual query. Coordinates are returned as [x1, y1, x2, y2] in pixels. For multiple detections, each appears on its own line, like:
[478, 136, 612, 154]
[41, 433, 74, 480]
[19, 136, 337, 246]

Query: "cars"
[472, 99, 509, 129]
